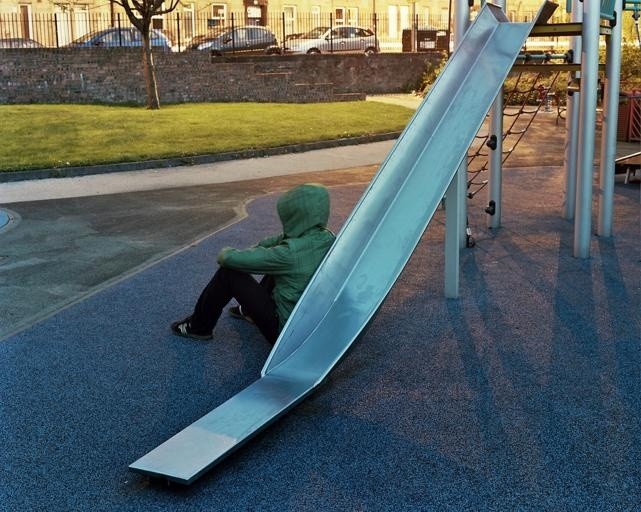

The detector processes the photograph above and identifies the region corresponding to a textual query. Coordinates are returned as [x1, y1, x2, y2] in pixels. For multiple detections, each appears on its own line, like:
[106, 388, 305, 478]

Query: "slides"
[127, 1, 560, 481]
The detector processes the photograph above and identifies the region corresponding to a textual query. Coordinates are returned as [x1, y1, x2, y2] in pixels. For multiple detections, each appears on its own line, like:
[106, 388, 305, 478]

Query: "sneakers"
[228, 304, 257, 325]
[170, 317, 213, 340]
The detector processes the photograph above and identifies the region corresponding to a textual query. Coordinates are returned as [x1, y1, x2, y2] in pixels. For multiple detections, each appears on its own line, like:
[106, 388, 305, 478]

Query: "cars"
[282, 24, 380, 56]
[54, 24, 174, 57]
[0, 38, 48, 52]
[184, 27, 280, 57]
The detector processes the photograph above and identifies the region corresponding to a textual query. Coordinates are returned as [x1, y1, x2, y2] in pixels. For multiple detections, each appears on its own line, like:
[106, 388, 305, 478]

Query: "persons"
[171, 183, 341, 347]
